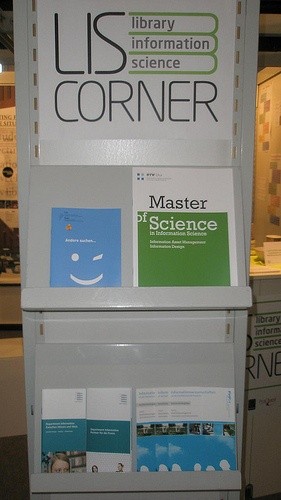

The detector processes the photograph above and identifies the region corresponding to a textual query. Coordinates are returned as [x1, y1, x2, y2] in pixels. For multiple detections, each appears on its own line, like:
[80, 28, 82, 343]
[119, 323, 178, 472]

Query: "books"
[40, 165, 239, 474]
